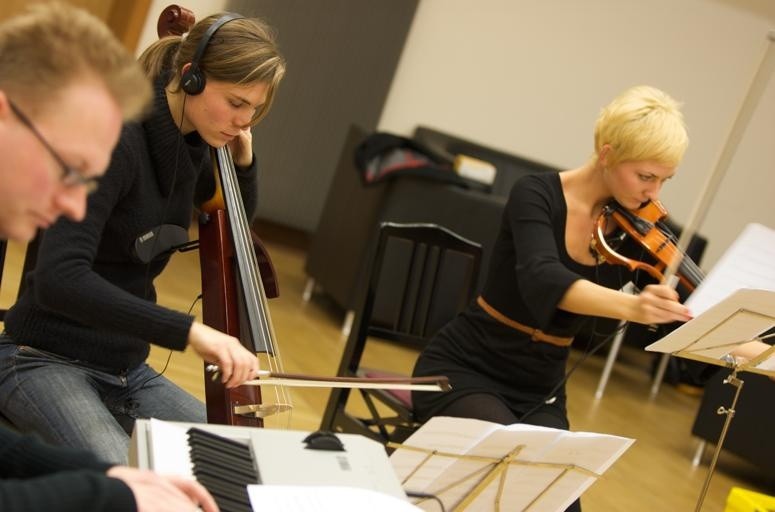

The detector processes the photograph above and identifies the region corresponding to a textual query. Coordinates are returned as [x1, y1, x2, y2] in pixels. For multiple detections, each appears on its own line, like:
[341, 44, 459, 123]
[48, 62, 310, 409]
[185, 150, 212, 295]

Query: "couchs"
[303, 122, 709, 393]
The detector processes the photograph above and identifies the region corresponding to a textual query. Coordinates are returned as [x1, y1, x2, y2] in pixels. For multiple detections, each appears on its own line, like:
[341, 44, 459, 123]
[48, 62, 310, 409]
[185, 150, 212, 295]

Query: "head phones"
[181, 11, 247, 97]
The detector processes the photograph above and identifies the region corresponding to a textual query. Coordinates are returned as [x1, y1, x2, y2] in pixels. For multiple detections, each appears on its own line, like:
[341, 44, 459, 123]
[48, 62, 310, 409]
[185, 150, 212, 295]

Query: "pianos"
[126, 417, 424, 511]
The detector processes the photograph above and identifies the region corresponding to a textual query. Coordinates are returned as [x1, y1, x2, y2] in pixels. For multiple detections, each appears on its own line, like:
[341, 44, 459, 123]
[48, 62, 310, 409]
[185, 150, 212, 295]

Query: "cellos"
[156, 4, 293, 427]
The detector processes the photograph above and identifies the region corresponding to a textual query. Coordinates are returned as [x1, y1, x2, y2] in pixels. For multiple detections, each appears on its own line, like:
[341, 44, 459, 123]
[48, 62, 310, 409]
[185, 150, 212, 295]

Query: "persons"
[2, 11, 285, 476]
[1, 1, 223, 512]
[410, 81, 692, 512]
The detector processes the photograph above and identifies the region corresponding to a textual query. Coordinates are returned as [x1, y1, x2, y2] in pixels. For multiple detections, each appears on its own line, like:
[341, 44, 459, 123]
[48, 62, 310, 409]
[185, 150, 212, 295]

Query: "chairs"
[691, 367, 775, 474]
[322, 222, 484, 445]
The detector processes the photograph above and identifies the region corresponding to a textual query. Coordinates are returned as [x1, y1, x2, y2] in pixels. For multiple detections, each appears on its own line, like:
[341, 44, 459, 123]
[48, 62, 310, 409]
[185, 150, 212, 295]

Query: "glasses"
[7, 98, 101, 193]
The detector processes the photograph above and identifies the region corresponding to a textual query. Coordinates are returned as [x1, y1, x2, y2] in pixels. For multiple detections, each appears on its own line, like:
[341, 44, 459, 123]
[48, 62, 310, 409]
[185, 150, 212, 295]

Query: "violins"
[590, 195, 708, 293]
